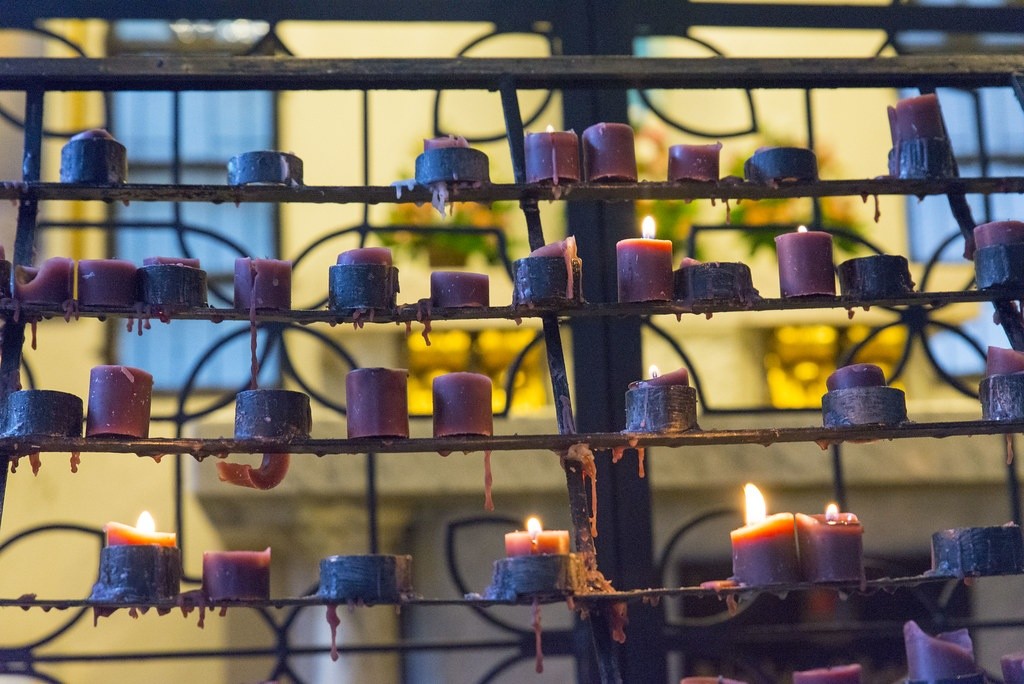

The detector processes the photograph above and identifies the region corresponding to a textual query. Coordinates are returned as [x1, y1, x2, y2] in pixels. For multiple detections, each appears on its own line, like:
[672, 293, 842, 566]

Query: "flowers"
[373, 160, 526, 262]
[730, 128, 866, 256]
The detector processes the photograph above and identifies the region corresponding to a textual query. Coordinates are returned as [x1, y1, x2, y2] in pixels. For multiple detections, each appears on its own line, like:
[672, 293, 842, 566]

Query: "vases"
[429, 242, 465, 268]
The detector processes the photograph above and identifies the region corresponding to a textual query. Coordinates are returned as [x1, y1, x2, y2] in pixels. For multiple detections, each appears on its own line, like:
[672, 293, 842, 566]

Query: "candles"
[529, 236, 577, 298]
[430, 271, 490, 309]
[904, 619, 976, 683]
[525, 124, 638, 185]
[504, 517, 570, 556]
[668, 139, 723, 183]
[618, 216, 701, 299]
[337, 247, 392, 265]
[235, 258, 293, 311]
[423, 135, 468, 151]
[345, 367, 410, 439]
[1002, 653, 1024, 684]
[795, 503, 863, 584]
[887, 94, 946, 145]
[974, 220, 1023, 249]
[107, 511, 177, 546]
[628, 364, 689, 390]
[202, 547, 271, 601]
[432, 371, 494, 437]
[72, 129, 114, 141]
[0, 245, 137, 310]
[142, 257, 201, 269]
[86, 365, 152, 439]
[731, 482, 800, 583]
[794, 662, 863, 684]
[826, 363, 887, 390]
[774, 225, 835, 298]
[985, 346, 1023, 376]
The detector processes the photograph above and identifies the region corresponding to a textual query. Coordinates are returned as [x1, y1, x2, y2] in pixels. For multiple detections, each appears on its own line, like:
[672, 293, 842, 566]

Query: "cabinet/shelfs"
[0, 0, 1024, 684]
[192, 265, 1024, 684]
